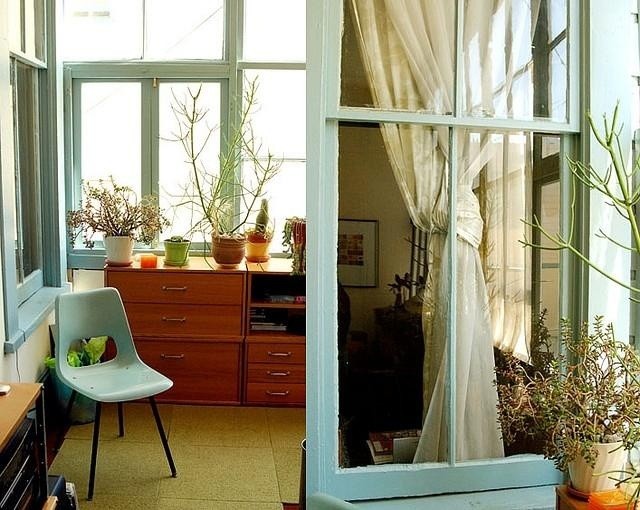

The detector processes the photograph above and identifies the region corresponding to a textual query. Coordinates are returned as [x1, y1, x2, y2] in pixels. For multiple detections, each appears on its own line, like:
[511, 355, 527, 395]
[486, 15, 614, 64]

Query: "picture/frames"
[337, 214, 382, 290]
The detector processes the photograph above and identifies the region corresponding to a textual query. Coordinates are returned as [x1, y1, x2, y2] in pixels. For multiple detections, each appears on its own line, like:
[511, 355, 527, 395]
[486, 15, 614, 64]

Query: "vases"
[101, 233, 137, 269]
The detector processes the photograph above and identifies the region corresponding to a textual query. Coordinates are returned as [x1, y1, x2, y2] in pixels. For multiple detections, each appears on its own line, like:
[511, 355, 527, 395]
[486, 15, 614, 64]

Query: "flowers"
[59, 173, 168, 251]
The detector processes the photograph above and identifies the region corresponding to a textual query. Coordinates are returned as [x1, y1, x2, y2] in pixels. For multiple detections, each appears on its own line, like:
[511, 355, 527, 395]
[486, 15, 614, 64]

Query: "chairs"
[53, 284, 180, 502]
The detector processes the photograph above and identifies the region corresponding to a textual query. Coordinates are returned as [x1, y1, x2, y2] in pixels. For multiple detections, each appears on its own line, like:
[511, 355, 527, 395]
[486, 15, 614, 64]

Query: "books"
[250, 308, 290, 333]
[366, 428, 422, 465]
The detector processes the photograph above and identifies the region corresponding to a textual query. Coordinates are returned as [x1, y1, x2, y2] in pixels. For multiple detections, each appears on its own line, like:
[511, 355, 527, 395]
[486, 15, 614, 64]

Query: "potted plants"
[243, 195, 274, 262]
[161, 234, 192, 267]
[171, 73, 285, 267]
[483, 308, 640, 501]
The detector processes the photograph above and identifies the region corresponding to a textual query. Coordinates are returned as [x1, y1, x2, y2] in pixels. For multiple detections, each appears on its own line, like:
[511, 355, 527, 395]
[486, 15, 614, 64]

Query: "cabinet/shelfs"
[549, 479, 640, 510]
[1, 378, 71, 510]
[243, 254, 308, 411]
[103, 253, 248, 409]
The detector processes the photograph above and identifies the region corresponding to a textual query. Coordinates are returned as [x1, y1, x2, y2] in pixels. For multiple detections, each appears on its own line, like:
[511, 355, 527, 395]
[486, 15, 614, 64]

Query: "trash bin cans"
[48, 359, 101, 426]
[298, 439, 306, 510]
